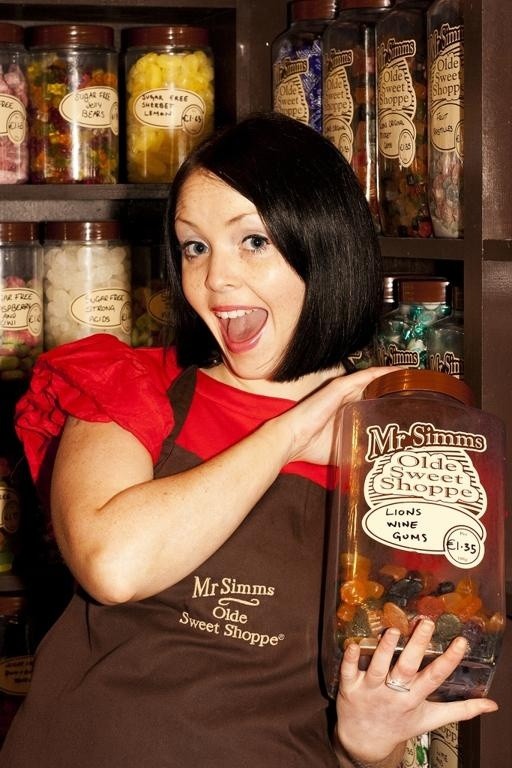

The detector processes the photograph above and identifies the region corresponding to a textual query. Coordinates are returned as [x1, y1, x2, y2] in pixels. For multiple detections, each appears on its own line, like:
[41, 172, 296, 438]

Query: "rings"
[385, 671, 414, 691]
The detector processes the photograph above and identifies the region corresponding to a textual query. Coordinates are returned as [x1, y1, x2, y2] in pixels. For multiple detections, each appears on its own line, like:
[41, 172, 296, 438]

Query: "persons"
[0, 103, 502, 765]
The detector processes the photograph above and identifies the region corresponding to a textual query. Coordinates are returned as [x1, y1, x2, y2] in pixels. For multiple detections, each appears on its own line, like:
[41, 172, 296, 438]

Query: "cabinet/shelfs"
[0, 0, 512, 768]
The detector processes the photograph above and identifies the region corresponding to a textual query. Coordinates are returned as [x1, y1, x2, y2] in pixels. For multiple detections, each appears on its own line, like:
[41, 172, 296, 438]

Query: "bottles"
[320, 371, 504, 699]
[1, 14, 223, 187]
[2, 222, 196, 375]
[259, 5, 472, 243]
[357, 269, 462, 389]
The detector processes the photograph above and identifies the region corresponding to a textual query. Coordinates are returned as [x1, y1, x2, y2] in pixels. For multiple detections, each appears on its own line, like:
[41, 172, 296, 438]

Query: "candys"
[272, 36, 466, 237]
[0, 50, 216, 186]
[332, 552, 507, 703]
[1, 237, 175, 383]
[354, 300, 450, 370]
